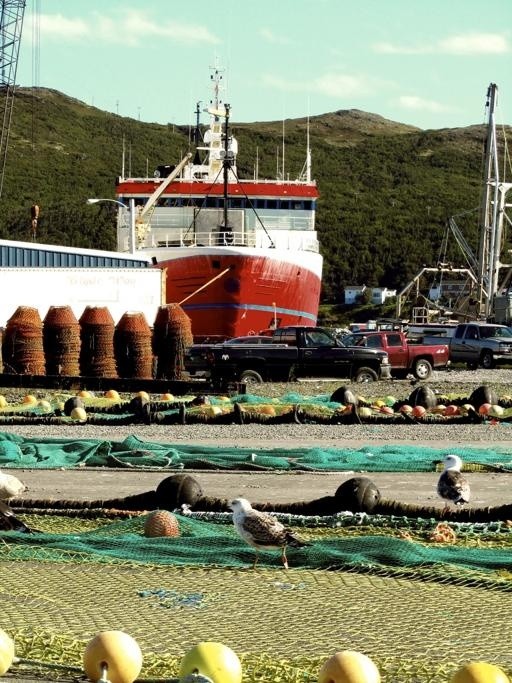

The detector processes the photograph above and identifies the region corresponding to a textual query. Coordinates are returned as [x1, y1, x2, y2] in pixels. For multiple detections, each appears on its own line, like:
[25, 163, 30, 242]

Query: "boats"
[114, 61, 324, 341]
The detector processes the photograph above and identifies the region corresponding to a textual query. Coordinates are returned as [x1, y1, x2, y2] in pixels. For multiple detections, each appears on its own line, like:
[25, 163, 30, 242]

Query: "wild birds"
[431, 452, 471, 508]
[220, 497, 315, 571]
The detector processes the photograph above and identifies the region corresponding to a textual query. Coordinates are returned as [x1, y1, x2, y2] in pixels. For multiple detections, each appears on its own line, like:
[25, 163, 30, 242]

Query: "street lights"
[88, 191, 137, 250]
